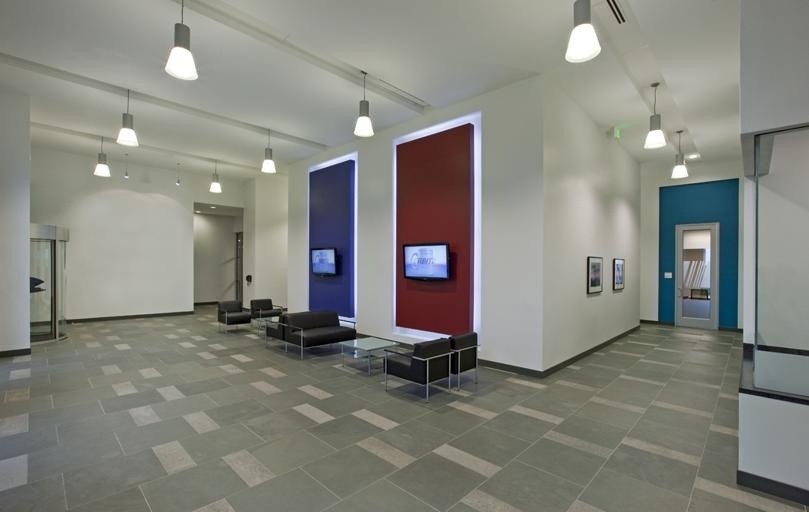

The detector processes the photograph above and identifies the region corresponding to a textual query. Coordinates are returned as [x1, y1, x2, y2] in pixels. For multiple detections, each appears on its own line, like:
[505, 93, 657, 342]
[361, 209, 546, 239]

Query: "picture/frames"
[586, 255, 604, 295]
[612, 257, 626, 291]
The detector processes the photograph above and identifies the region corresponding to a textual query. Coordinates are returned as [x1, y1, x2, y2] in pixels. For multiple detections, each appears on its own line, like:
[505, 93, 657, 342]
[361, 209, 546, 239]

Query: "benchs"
[284, 310, 358, 361]
[264, 313, 286, 348]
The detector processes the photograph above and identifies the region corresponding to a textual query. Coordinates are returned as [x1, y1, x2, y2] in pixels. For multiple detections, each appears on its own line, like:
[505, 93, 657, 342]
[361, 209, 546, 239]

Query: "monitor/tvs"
[403, 243, 450, 280]
[310, 247, 336, 276]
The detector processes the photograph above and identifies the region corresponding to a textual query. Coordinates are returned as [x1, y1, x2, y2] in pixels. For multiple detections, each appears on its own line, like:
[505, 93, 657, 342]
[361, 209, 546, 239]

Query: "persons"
[591, 264, 597, 286]
[594, 268, 600, 286]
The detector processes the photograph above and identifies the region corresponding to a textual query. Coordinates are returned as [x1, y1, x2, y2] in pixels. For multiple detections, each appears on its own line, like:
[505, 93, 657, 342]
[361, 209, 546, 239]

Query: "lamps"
[207, 159, 224, 195]
[92, 137, 111, 179]
[121, 161, 132, 181]
[174, 162, 183, 188]
[643, 82, 667, 151]
[352, 71, 376, 140]
[668, 127, 691, 181]
[113, 86, 143, 151]
[561, 0, 603, 66]
[161, 0, 199, 86]
[259, 125, 277, 176]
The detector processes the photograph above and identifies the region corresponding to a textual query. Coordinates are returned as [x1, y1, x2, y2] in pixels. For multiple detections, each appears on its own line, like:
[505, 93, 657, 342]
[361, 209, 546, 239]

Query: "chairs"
[250, 298, 283, 336]
[448, 330, 481, 391]
[383, 337, 455, 403]
[215, 298, 254, 335]
